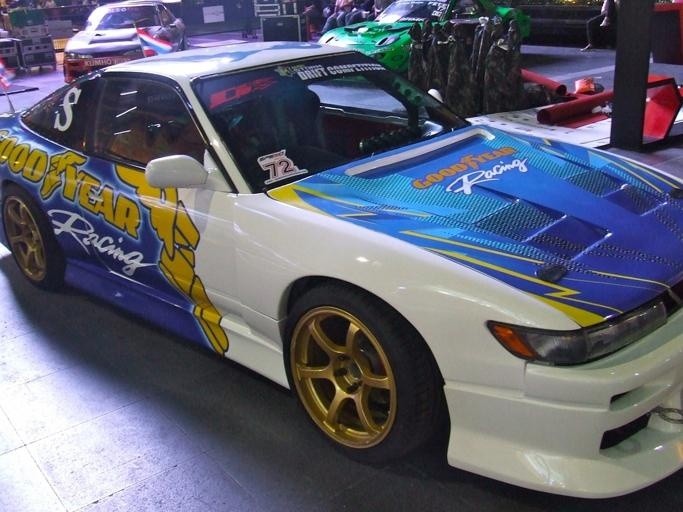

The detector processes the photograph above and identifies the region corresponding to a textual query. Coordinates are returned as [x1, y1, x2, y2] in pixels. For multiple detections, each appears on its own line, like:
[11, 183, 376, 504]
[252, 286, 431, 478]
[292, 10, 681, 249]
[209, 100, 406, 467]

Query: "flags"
[136, 28, 173, 57]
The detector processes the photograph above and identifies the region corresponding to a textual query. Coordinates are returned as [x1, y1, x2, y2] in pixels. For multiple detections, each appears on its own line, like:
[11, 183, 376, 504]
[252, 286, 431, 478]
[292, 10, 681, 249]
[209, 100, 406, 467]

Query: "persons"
[319, 0, 392, 37]
[580, 1, 617, 55]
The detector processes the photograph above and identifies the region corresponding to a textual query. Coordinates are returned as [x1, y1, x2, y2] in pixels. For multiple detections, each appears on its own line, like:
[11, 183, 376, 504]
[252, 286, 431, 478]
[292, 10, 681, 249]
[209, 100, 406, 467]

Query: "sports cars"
[2, 43, 683, 498]
[319, 1, 535, 82]
[62, 0, 189, 85]
